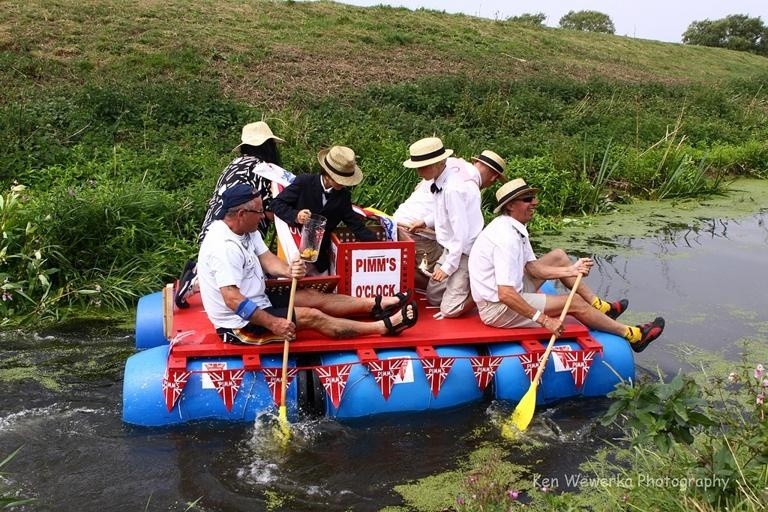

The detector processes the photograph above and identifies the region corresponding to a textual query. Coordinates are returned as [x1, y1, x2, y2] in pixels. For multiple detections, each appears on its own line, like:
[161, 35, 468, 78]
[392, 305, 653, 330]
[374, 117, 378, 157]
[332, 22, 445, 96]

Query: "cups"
[298, 213, 327, 264]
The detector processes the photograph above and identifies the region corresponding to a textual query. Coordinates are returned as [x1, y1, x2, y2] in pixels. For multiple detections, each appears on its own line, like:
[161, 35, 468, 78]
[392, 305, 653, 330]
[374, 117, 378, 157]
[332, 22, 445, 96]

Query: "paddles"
[253, 162, 435, 236]
[510, 260, 589, 430]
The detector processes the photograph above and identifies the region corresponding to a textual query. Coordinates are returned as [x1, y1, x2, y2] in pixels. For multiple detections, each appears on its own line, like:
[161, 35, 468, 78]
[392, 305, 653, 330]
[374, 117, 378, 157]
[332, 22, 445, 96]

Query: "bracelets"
[542, 316, 548, 328]
[531, 311, 542, 322]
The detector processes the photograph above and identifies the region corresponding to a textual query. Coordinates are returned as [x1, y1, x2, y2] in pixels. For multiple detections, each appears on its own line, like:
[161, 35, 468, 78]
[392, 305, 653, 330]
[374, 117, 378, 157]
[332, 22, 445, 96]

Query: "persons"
[467, 177, 665, 351]
[272, 146, 377, 276]
[176, 121, 287, 309]
[393, 150, 507, 287]
[196, 184, 416, 347]
[401, 135, 485, 319]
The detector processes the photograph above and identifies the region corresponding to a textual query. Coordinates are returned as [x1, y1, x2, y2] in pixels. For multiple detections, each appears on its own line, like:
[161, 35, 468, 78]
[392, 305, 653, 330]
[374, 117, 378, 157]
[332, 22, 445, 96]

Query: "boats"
[156, 391, 625, 512]
[118, 270, 642, 434]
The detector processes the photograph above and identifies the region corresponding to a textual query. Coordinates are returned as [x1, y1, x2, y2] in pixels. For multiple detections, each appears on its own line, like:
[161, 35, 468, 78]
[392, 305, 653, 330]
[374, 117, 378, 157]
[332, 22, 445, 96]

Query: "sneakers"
[605, 299, 628, 320]
[175, 260, 199, 308]
[630, 317, 665, 353]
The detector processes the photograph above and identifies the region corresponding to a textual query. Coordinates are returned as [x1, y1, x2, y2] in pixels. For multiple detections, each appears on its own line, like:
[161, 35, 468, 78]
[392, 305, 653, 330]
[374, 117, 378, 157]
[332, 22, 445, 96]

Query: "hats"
[232, 122, 286, 152]
[471, 149, 507, 183]
[493, 178, 538, 213]
[216, 184, 260, 220]
[403, 138, 453, 168]
[317, 146, 363, 186]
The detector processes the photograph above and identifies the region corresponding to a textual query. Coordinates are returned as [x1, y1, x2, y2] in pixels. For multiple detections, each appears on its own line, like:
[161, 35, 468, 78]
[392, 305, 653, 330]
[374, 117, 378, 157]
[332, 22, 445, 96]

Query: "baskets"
[330, 225, 415, 305]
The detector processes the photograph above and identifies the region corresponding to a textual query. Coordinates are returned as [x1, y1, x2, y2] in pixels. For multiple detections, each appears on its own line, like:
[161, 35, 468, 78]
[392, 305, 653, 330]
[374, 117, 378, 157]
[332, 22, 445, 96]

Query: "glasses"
[513, 196, 536, 202]
[237, 208, 264, 215]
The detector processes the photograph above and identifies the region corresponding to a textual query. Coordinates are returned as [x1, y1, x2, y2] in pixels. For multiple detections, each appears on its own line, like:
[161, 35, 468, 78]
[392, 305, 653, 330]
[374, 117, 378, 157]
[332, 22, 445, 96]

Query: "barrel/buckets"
[487, 331, 635, 403]
[132, 291, 169, 347]
[121, 344, 298, 429]
[318, 346, 485, 418]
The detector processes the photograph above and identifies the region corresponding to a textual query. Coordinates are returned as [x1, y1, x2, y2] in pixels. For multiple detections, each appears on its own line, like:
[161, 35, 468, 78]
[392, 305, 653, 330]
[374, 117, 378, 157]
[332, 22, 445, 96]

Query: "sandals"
[372, 288, 411, 319]
[382, 302, 418, 335]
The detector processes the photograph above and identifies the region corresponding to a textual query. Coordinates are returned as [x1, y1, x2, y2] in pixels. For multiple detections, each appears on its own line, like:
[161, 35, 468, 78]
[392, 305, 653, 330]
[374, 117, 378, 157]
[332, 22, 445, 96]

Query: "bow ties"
[325, 193, 330, 200]
[431, 183, 442, 194]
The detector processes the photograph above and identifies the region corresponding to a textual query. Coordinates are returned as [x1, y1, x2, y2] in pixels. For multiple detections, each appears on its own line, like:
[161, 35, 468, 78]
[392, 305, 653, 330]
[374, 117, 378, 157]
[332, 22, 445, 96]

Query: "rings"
[288, 332, 292, 337]
[559, 329, 562, 332]
